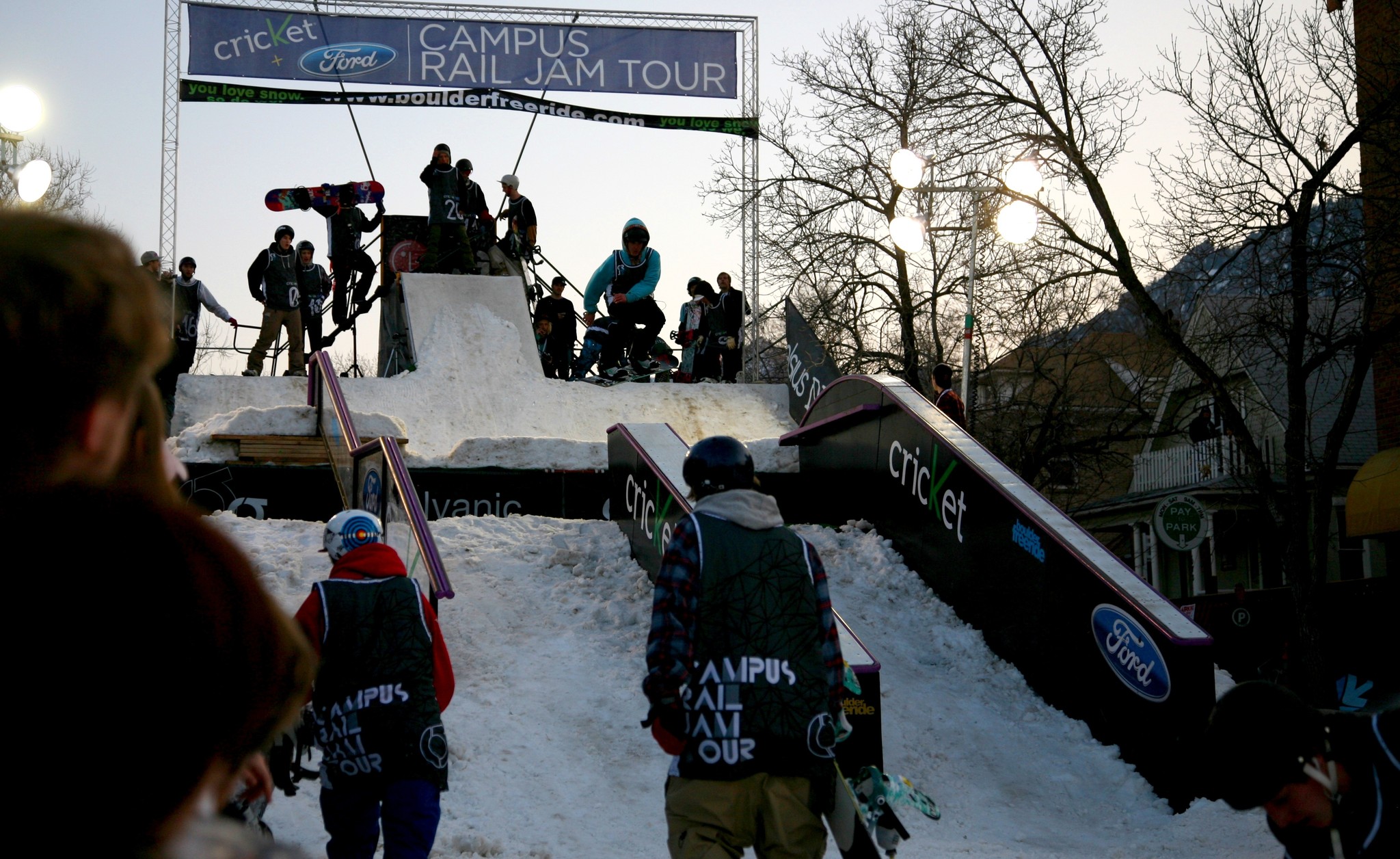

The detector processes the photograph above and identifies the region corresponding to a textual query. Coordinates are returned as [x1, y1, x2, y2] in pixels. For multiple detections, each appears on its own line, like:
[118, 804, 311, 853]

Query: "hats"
[1202, 406, 1211, 413]
[141, 251, 165, 264]
[552, 276, 567, 286]
[694, 295, 704, 301]
[932, 363, 954, 389]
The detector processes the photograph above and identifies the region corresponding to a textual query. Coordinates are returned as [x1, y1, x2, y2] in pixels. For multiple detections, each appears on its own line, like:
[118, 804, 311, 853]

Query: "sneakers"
[294, 368, 306, 375]
[243, 370, 259, 376]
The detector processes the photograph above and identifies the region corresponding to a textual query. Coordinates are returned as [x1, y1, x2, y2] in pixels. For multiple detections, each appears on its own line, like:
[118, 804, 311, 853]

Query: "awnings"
[1345, 447, 1400, 540]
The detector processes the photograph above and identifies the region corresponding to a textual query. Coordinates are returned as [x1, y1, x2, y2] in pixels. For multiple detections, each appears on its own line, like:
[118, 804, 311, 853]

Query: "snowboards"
[822, 757, 881, 859]
[265, 180, 384, 212]
[578, 352, 679, 387]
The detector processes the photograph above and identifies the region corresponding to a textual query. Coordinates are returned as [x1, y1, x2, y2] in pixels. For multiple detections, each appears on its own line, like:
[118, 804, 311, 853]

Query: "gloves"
[726, 336, 736, 350]
[376, 200, 383, 208]
[651, 717, 687, 757]
[321, 183, 334, 191]
[696, 335, 704, 347]
[268, 733, 301, 798]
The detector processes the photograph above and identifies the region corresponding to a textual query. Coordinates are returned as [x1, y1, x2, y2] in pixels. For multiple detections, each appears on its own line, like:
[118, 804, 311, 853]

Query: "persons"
[641, 436, 845, 859]
[419, 143, 752, 383]
[0, 183, 385, 859]
[293, 508, 456, 859]
[1205, 681, 1400, 859]
[931, 363, 966, 431]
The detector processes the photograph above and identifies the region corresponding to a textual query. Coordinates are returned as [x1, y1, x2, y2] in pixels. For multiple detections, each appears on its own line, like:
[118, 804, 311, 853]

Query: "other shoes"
[352, 297, 368, 304]
[333, 317, 347, 324]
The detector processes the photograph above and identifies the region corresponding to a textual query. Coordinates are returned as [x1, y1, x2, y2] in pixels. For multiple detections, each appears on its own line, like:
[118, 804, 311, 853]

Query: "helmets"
[300, 242, 314, 254]
[682, 435, 755, 493]
[455, 159, 473, 170]
[274, 225, 294, 242]
[434, 143, 451, 164]
[624, 227, 648, 245]
[178, 257, 197, 271]
[687, 277, 701, 293]
[497, 174, 520, 190]
[316, 510, 383, 560]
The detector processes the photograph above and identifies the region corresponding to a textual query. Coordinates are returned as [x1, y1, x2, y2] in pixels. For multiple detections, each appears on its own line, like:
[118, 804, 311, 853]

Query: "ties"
[718, 294, 725, 316]
